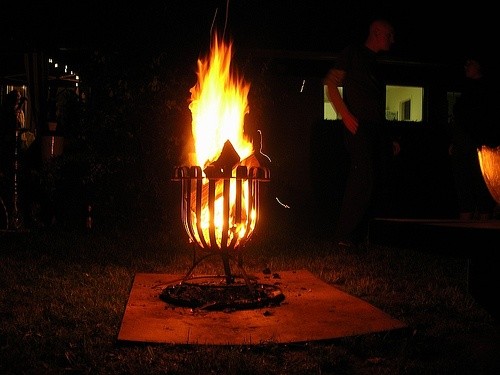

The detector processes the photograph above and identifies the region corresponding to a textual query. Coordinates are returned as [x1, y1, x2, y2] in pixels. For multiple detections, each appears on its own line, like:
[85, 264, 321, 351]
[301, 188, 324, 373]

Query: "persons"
[433, 51, 498, 214]
[327, 16, 414, 247]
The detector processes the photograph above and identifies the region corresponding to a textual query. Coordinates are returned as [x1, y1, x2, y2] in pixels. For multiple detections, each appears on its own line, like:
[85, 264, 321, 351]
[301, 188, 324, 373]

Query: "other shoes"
[331, 236, 358, 252]
